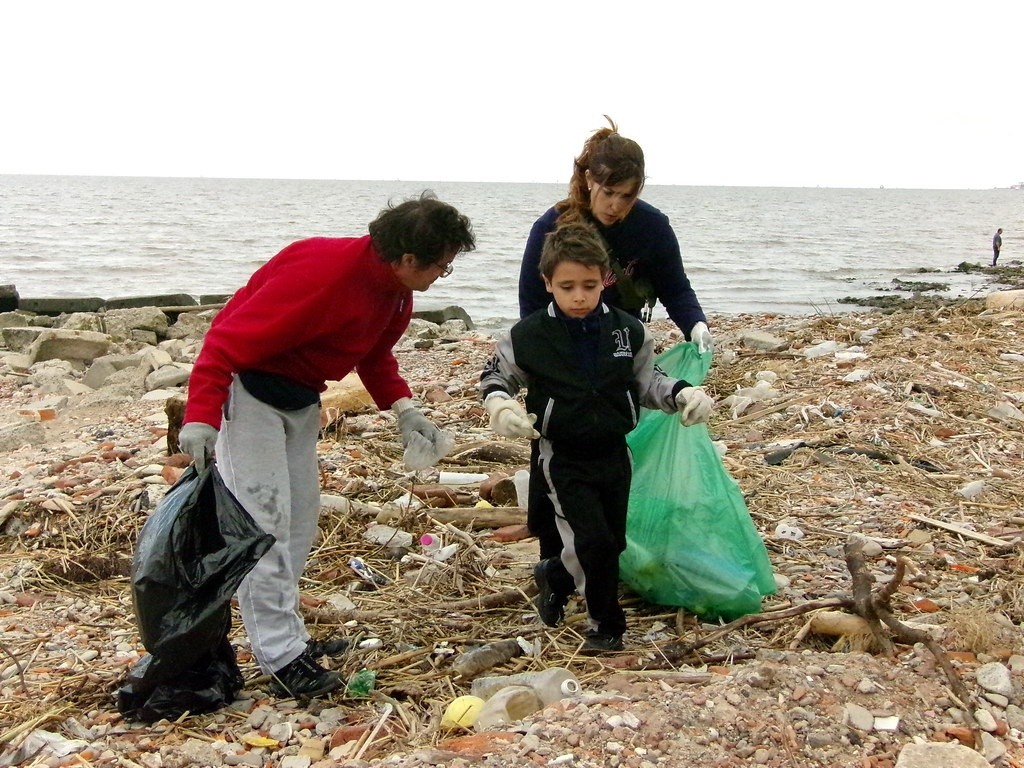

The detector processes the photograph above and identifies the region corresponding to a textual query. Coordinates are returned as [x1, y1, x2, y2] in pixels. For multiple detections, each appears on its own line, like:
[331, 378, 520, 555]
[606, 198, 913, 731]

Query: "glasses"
[433, 261, 453, 279]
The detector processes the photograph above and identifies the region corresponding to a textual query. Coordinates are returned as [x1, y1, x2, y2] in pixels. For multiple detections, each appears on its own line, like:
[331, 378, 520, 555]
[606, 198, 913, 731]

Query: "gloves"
[398, 407, 443, 450]
[690, 322, 711, 355]
[179, 422, 218, 479]
[674, 385, 711, 427]
[484, 395, 541, 441]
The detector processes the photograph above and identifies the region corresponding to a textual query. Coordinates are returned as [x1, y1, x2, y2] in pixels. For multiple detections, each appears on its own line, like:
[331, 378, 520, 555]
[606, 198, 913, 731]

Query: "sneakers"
[304, 635, 349, 657]
[534, 560, 567, 625]
[578, 635, 625, 656]
[272, 655, 342, 697]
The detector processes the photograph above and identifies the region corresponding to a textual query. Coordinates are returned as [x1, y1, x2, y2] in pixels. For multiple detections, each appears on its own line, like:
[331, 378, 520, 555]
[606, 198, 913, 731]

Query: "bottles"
[439, 471, 490, 485]
[961, 480, 986, 498]
[833, 325, 880, 362]
[805, 339, 840, 359]
[732, 371, 780, 399]
[321, 491, 386, 519]
[468, 666, 583, 709]
[416, 532, 447, 553]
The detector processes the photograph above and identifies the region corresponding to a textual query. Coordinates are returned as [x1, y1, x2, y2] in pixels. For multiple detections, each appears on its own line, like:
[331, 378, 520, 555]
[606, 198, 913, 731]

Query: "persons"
[518, 128, 712, 562]
[993, 228, 1002, 266]
[179, 197, 478, 700]
[643, 298, 656, 323]
[479, 222, 713, 654]
